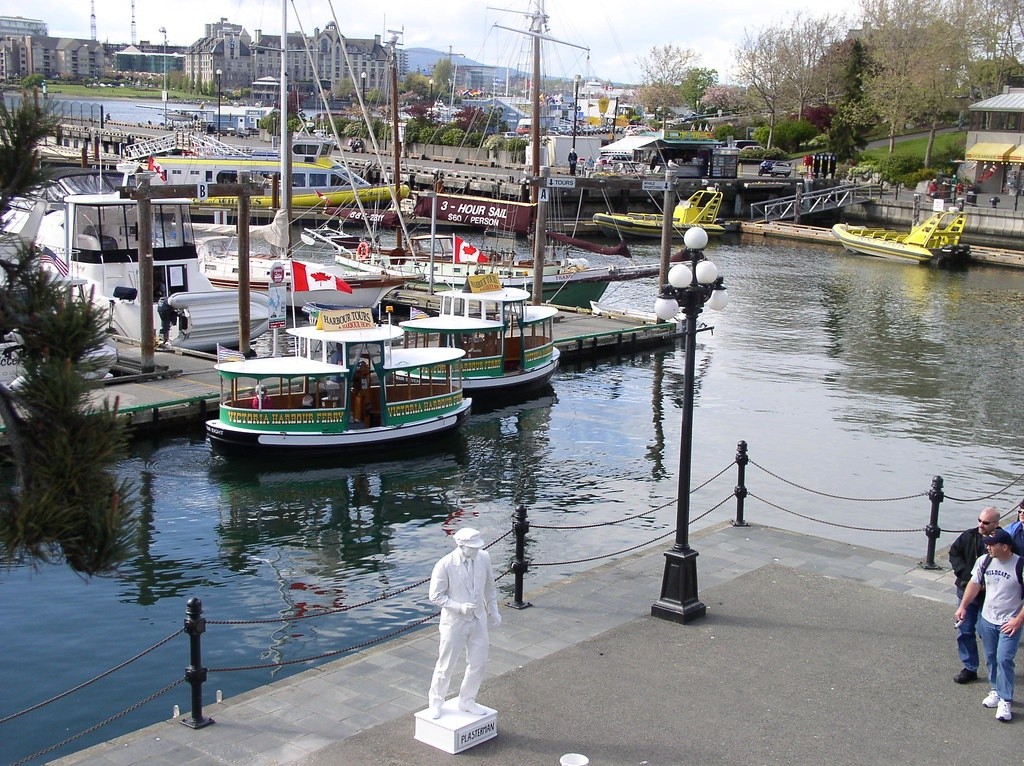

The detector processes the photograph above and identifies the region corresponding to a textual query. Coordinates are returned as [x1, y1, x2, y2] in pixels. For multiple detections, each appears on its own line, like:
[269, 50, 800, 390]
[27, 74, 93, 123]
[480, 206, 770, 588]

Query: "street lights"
[650, 227, 728, 625]
[361, 72, 366, 128]
[429, 78, 434, 128]
[216, 68, 223, 140]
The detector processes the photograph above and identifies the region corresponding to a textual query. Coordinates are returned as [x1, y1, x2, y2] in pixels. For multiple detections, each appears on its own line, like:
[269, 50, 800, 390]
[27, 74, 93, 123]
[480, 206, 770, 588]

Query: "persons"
[348, 138, 365, 153]
[253, 384, 273, 409]
[298, 395, 315, 409]
[584, 155, 594, 177]
[330, 342, 343, 407]
[955, 527, 1024, 720]
[926, 179, 938, 201]
[1003, 499, 1024, 559]
[949, 507, 1019, 683]
[428, 527, 502, 720]
[568, 147, 578, 176]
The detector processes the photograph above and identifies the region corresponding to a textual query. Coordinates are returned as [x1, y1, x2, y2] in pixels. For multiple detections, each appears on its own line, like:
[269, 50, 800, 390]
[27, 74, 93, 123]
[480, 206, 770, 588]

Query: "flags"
[411, 307, 430, 319]
[455, 235, 488, 263]
[314, 188, 332, 205]
[41, 247, 69, 277]
[293, 262, 352, 293]
[148, 156, 167, 182]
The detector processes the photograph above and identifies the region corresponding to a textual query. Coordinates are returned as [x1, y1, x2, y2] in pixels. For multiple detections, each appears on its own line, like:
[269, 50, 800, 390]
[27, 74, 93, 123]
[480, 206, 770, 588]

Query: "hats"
[981, 529, 1013, 546]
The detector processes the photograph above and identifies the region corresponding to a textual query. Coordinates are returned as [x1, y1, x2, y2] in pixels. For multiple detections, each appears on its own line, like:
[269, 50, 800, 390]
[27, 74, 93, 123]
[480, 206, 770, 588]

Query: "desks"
[321, 397, 339, 408]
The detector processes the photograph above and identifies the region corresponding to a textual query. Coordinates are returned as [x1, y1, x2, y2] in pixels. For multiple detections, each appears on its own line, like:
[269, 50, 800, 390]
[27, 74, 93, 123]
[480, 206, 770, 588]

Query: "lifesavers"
[356, 241, 371, 262]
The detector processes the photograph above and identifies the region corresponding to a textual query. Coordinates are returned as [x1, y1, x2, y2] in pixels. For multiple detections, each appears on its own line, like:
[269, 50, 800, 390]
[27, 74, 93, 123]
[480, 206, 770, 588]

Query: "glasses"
[977, 518, 996, 525]
[1018, 511, 1024, 514]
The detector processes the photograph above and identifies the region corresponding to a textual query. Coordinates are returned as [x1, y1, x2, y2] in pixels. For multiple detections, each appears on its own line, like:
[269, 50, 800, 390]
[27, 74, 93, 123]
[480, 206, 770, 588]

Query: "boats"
[1, 0, 711, 350]
[205, 308, 472, 448]
[832, 207, 972, 265]
[593, 186, 726, 239]
[393, 274, 560, 389]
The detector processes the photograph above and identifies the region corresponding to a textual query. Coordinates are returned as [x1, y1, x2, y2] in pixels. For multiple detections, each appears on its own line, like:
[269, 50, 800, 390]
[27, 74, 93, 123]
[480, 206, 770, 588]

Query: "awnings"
[966, 143, 1016, 161]
[1010, 144, 1024, 163]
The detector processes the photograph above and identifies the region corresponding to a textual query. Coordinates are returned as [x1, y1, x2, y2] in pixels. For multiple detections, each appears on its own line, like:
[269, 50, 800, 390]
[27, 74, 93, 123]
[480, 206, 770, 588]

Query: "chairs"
[575, 158, 661, 178]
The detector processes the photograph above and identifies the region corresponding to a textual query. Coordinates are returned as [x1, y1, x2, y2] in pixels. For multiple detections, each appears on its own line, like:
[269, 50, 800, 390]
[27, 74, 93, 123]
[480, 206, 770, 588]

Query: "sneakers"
[982, 691, 999, 707]
[994, 700, 1012, 720]
[953, 668, 978, 683]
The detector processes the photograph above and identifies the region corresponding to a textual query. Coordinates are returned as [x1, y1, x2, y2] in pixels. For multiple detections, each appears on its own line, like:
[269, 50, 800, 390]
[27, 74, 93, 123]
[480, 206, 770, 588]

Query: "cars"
[758, 160, 791, 177]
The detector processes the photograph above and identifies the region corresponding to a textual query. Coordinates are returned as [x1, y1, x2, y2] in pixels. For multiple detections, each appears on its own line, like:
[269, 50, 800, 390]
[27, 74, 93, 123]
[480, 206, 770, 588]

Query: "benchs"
[611, 213, 682, 226]
[849, 229, 909, 244]
[505, 357, 521, 363]
